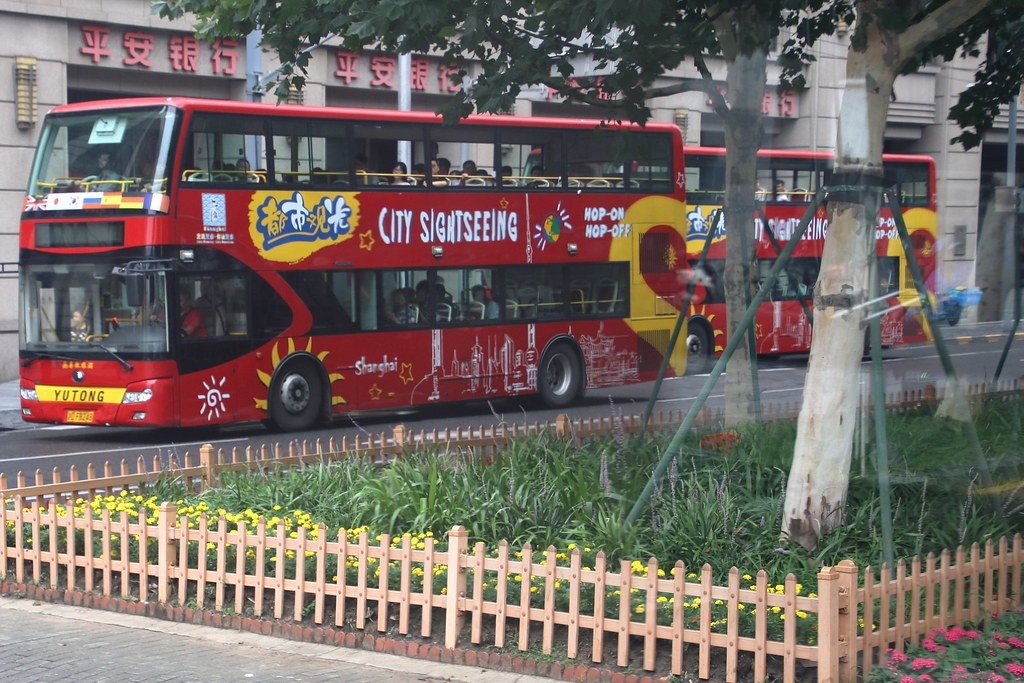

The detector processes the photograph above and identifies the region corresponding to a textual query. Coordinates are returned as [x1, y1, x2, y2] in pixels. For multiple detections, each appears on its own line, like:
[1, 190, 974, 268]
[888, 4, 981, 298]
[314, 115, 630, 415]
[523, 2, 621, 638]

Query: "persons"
[149, 289, 208, 337]
[471, 285, 499, 320]
[413, 158, 495, 188]
[775, 180, 787, 201]
[501, 165, 513, 184]
[69, 309, 87, 343]
[212, 158, 256, 183]
[82, 152, 120, 192]
[384, 279, 453, 326]
[526, 166, 545, 188]
[388, 162, 410, 185]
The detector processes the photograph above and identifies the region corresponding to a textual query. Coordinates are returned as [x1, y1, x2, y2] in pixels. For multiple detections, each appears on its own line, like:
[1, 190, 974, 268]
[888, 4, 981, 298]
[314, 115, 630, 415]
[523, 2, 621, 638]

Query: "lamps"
[12, 55, 38, 132]
[673, 108, 690, 146]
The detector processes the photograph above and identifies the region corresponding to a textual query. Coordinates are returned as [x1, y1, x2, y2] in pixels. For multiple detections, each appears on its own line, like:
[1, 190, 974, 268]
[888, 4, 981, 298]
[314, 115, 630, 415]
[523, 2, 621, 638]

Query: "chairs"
[213, 174, 234, 182]
[78, 175, 98, 192]
[469, 301, 486, 320]
[570, 279, 590, 313]
[590, 277, 619, 313]
[506, 300, 517, 318]
[436, 303, 452, 322]
[777, 282, 816, 296]
[235, 173, 260, 183]
[888, 189, 905, 204]
[413, 304, 420, 324]
[788, 188, 817, 201]
[406, 176, 641, 185]
[506, 280, 518, 300]
[755, 187, 765, 201]
[188, 173, 213, 181]
[569, 289, 585, 315]
[519, 288, 541, 319]
[121, 176, 129, 193]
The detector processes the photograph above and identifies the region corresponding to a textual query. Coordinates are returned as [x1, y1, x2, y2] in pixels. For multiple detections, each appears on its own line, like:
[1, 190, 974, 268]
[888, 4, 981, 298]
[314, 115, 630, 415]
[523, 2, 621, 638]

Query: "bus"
[516, 144, 940, 377]
[17, 96, 689, 436]
